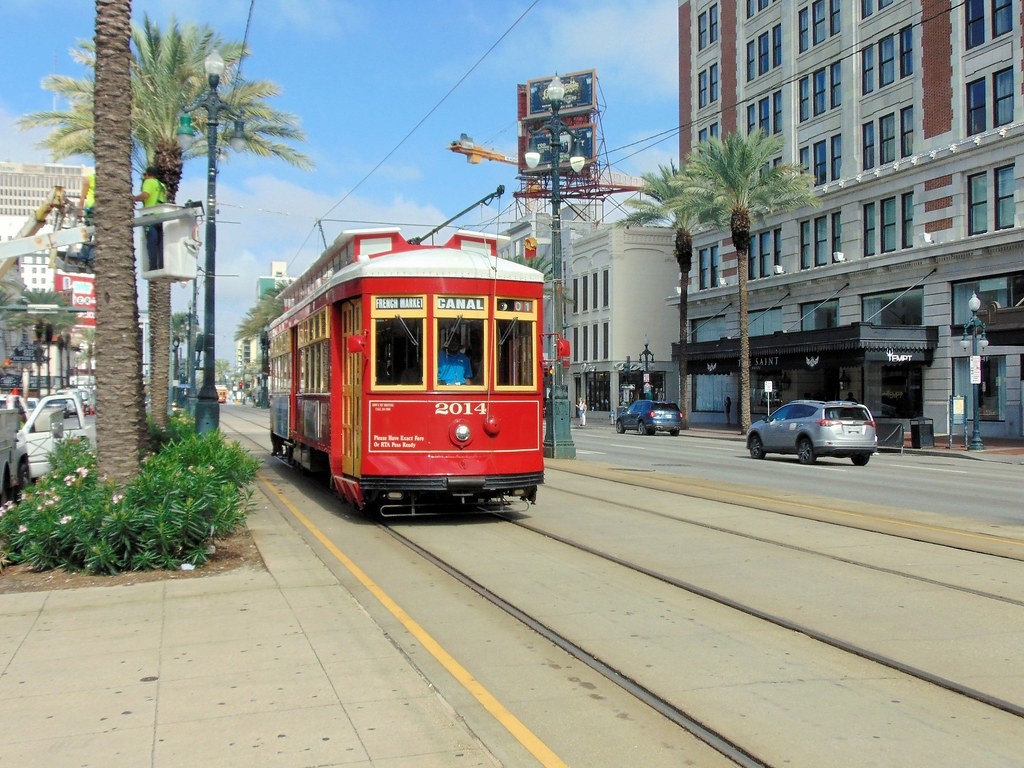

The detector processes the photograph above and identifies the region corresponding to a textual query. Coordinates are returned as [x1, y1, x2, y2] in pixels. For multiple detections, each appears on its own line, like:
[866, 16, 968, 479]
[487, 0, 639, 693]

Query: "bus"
[266, 184, 571, 519]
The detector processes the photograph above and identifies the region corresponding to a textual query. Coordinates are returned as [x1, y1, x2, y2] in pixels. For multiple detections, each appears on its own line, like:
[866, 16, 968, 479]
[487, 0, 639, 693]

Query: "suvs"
[615, 399, 684, 436]
[746, 400, 878, 467]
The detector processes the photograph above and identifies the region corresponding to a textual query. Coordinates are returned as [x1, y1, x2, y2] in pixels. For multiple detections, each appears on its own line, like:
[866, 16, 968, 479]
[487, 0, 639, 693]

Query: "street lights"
[522, 73, 588, 459]
[258, 324, 272, 409]
[180, 266, 206, 416]
[959, 290, 990, 450]
[638, 334, 656, 400]
[171, 48, 251, 440]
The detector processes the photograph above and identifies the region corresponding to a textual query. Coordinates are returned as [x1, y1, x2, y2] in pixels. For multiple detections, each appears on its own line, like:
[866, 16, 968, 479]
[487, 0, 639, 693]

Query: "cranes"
[447, 122, 654, 223]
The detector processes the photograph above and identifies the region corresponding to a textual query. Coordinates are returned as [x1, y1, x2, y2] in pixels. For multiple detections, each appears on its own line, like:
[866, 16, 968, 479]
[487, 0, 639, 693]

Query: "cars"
[0, 387, 94, 506]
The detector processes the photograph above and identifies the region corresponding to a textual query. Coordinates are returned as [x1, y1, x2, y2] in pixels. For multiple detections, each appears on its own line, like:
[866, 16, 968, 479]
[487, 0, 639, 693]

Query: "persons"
[77, 174, 95, 269]
[135, 167, 168, 271]
[419, 332, 473, 386]
[844, 392, 858, 404]
[724, 397, 731, 425]
[576, 398, 587, 427]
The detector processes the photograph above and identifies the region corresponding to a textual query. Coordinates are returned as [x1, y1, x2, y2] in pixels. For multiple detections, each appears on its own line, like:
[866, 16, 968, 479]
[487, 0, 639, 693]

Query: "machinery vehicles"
[0, 181, 205, 286]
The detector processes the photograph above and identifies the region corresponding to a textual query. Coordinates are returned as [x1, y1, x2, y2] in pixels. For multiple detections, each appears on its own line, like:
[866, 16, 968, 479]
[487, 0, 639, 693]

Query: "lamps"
[717, 278, 727, 287]
[774, 331, 783, 337]
[832, 251, 846, 262]
[720, 337, 727, 343]
[919, 233, 934, 245]
[838, 369, 851, 391]
[781, 372, 792, 391]
[673, 287, 681, 295]
[772, 264, 786, 275]
[851, 322, 860, 329]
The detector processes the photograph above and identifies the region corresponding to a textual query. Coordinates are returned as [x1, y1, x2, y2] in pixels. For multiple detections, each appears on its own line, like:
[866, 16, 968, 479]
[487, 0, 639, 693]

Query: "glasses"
[450, 339, 459, 343]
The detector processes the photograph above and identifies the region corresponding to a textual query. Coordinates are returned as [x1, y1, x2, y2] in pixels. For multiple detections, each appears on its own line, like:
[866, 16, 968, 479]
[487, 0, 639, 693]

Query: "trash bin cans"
[910, 417, 935, 450]
